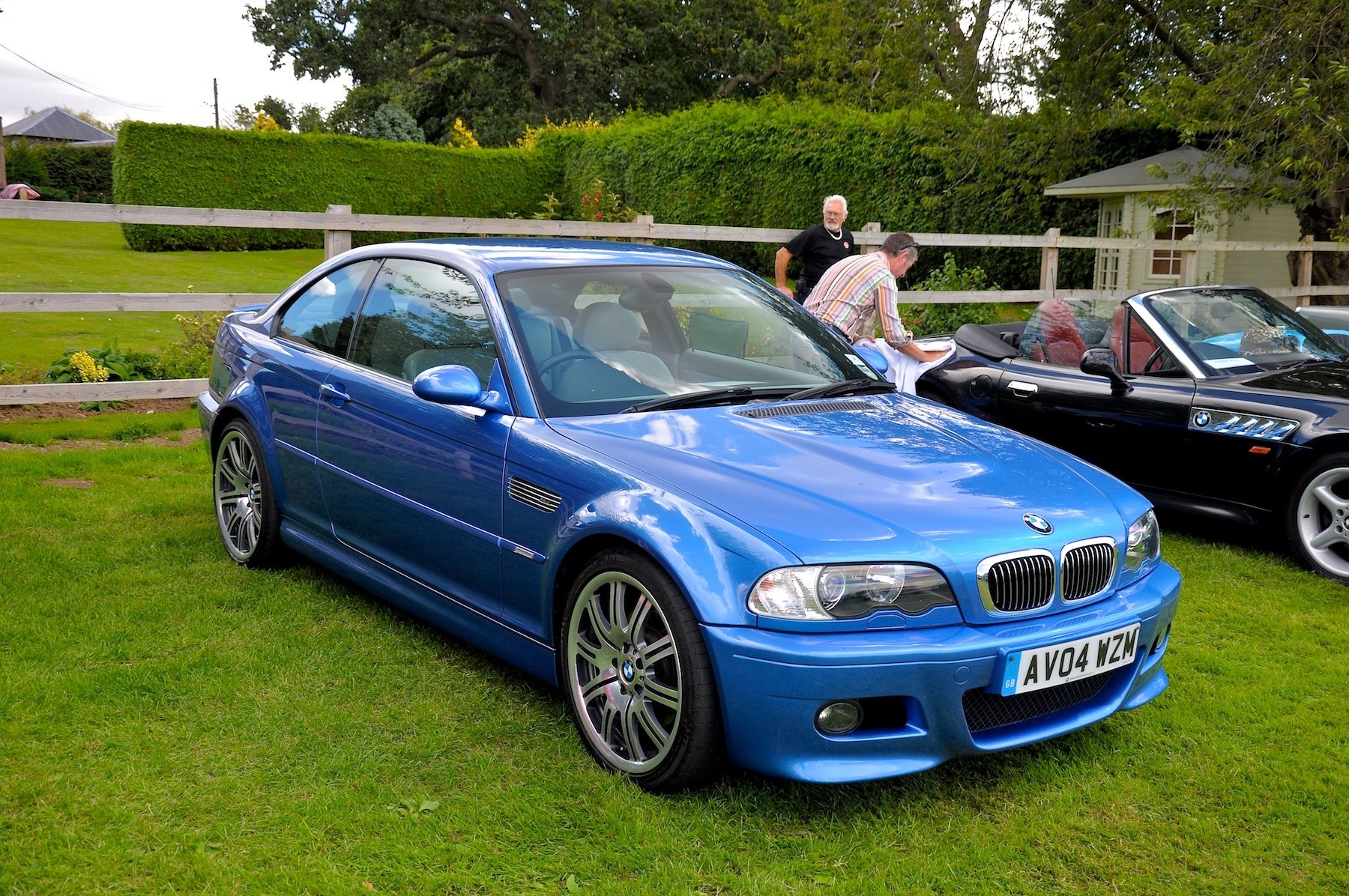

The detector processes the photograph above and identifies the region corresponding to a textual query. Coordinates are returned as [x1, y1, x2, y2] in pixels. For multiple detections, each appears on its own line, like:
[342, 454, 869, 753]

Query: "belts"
[826, 324, 850, 341]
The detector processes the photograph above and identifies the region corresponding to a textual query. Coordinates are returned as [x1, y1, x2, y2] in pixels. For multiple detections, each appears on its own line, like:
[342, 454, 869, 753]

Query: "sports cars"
[867, 285, 1349, 590]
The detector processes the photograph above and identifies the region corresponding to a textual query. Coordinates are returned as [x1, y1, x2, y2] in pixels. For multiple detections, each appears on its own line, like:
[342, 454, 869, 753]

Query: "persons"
[775, 195, 854, 305]
[792, 232, 951, 380]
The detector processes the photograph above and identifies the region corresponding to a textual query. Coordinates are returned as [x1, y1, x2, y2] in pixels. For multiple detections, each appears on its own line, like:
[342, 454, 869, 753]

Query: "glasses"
[900, 242, 920, 251]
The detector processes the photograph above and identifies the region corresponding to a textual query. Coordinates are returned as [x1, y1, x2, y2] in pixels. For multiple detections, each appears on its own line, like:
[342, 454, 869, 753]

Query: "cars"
[192, 235, 1188, 795]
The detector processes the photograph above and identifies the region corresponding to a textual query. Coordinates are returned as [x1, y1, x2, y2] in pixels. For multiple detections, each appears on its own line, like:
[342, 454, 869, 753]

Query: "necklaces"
[825, 226, 842, 240]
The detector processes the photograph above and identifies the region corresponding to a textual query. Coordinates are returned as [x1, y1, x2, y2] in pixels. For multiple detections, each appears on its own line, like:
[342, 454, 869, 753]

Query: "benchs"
[354, 314, 574, 375]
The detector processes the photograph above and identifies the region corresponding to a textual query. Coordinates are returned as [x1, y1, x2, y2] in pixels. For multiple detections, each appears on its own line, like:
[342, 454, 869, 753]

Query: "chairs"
[401, 310, 495, 395]
[1026, 299, 1090, 368]
[1113, 303, 1164, 375]
[553, 301, 672, 400]
